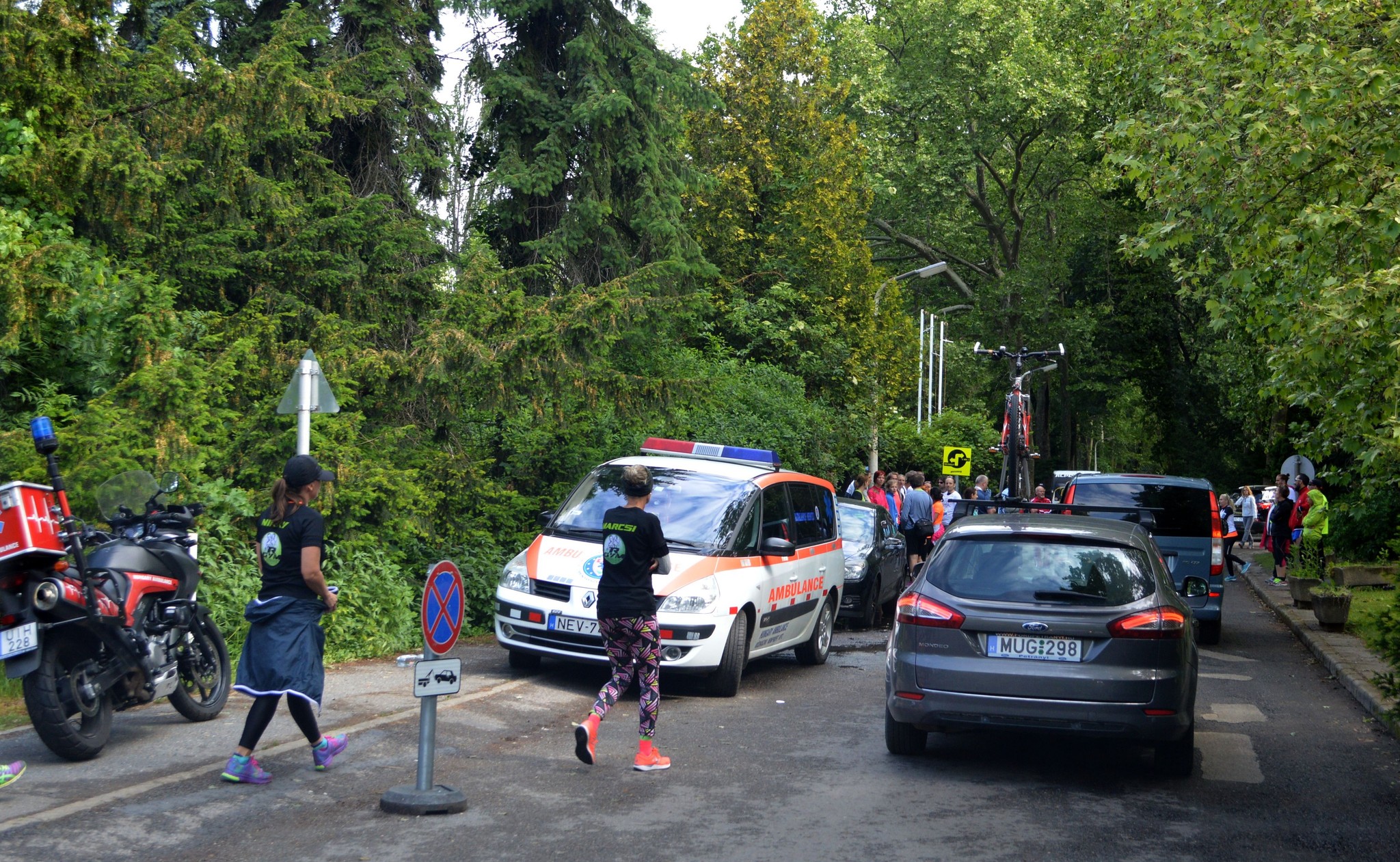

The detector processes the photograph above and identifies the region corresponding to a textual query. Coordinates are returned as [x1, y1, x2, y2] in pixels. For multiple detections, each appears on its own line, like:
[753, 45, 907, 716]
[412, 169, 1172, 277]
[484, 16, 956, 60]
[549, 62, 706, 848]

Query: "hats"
[1308, 479, 1322, 489]
[283, 454, 334, 486]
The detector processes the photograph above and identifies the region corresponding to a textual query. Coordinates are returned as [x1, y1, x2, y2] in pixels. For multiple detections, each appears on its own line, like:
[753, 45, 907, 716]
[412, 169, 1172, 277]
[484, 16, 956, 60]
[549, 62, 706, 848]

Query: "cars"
[883, 496, 1210, 779]
[834, 497, 909, 630]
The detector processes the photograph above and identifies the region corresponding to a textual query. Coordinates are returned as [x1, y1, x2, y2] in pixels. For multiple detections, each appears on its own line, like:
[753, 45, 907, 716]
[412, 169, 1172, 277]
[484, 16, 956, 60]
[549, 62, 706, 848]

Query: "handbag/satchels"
[914, 518, 934, 538]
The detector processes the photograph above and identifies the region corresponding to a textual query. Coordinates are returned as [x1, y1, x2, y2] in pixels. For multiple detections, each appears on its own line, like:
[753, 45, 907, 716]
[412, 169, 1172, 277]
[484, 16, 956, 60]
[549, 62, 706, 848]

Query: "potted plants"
[1310, 577, 1355, 632]
[1288, 537, 1327, 610]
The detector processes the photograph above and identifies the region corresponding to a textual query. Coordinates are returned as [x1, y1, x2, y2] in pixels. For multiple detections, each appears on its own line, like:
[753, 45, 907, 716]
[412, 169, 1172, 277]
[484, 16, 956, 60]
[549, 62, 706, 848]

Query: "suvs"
[1229, 485, 1277, 538]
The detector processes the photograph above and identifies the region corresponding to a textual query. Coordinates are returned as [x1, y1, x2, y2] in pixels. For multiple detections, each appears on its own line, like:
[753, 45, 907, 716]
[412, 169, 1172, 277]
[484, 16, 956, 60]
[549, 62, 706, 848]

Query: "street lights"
[866, 261, 974, 489]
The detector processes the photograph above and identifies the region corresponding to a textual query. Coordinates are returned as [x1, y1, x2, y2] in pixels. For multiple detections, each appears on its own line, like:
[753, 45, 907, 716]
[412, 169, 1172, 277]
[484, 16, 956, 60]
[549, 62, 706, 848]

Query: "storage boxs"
[0, 481, 67, 576]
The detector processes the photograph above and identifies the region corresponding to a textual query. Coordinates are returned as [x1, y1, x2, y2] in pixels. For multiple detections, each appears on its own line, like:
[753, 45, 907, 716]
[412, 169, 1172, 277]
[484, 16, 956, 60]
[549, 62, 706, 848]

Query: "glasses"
[924, 481, 931, 483]
[1295, 478, 1301, 481]
[938, 482, 944, 484]
[1244, 489, 1248, 491]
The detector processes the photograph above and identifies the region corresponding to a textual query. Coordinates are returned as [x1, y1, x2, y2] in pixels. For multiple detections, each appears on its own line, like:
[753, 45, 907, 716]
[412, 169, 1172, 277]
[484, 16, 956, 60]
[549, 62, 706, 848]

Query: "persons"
[0, 761, 26, 789]
[573, 465, 670, 771]
[1217, 474, 1329, 586]
[845, 470, 1053, 584]
[221, 455, 348, 784]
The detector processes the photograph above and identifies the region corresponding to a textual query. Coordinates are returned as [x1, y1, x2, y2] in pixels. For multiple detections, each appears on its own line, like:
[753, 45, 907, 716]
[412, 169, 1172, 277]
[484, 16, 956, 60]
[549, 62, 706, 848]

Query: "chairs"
[1086, 557, 1128, 589]
[979, 553, 1018, 578]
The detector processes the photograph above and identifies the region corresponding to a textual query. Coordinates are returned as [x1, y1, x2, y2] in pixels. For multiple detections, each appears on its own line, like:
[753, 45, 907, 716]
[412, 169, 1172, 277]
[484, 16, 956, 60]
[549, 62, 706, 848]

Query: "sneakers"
[633, 747, 671, 771]
[1265, 579, 1274, 583]
[1241, 561, 1251, 574]
[1268, 578, 1281, 585]
[1273, 579, 1287, 586]
[0, 760, 27, 788]
[1224, 575, 1236, 581]
[575, 720, 598, 765]
[222, 755, 272, 784]
[312, 733, 348, 770]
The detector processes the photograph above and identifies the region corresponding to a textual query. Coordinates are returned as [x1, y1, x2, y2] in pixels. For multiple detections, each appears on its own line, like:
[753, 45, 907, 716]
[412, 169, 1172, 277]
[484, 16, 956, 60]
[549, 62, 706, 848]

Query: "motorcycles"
[0, 416, 231, 763]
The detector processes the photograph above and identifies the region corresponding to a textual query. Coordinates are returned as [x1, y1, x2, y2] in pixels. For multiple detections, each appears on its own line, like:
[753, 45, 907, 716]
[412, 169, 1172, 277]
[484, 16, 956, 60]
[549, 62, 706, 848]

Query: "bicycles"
[973, 340, 1065, 498]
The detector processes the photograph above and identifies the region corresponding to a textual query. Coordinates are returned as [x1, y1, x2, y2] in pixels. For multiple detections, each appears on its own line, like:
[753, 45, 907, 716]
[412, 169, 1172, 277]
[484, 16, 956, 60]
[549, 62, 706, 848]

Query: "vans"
[1053, 471, 1233, 648]
[494, 436, 842, 698]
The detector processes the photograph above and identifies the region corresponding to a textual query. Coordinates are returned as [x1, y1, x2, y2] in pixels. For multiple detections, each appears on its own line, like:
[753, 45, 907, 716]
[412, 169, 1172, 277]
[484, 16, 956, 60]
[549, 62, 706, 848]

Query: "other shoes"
[1247, 547, 1254, 549]
[1239, 543, 1243, 548]
